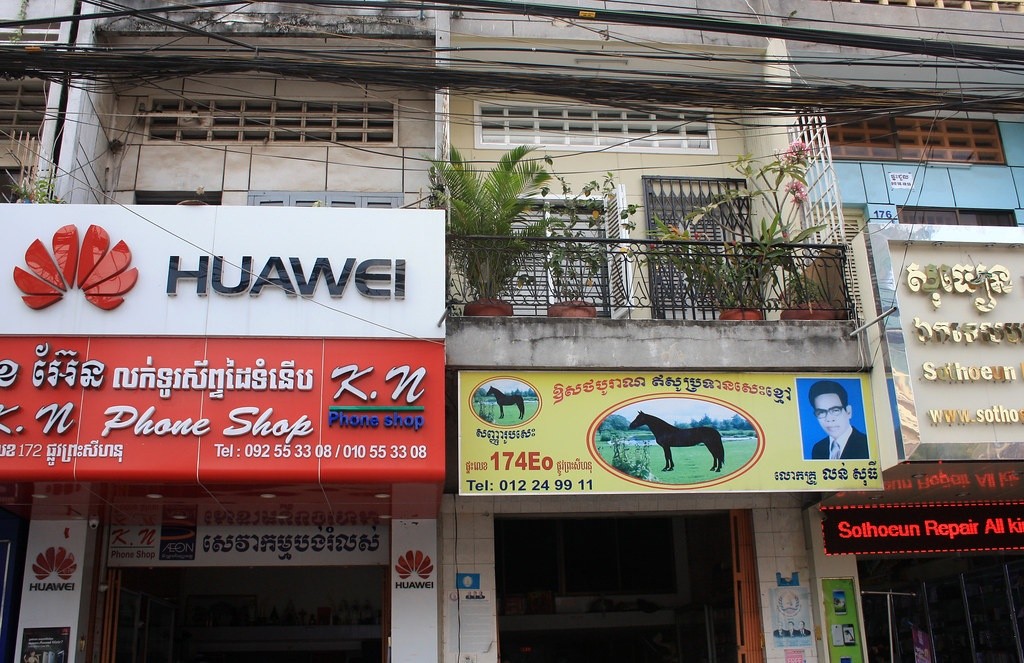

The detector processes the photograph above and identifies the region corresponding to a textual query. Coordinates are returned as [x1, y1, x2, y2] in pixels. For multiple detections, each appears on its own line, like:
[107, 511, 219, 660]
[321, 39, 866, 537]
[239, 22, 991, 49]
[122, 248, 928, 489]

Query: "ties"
[831, 441, 840, 459]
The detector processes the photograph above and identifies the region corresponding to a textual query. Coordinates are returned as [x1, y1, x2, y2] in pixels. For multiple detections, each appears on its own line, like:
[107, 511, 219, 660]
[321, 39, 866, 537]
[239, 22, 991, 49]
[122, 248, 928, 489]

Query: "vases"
[781, 301, 836, 320]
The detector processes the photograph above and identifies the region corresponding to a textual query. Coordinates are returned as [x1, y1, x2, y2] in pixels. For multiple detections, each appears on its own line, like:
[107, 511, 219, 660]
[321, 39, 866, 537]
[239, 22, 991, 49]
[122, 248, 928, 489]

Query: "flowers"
[685, 142, 836, 307]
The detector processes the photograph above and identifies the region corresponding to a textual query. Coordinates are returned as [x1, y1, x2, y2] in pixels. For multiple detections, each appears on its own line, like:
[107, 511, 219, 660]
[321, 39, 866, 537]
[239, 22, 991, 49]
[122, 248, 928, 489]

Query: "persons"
[808, 380, 870, 459]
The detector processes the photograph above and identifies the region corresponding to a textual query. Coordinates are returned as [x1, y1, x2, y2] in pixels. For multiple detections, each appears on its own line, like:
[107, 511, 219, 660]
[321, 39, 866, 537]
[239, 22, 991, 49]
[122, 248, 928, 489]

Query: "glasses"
[814, 406, 844, 419]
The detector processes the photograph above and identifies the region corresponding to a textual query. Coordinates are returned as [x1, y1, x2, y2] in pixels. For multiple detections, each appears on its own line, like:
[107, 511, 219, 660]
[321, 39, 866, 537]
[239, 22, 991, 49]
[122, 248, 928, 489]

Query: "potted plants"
[644, 183, 828, 321]
[424, 144, 557, 318]
[517, 156, 637, 318]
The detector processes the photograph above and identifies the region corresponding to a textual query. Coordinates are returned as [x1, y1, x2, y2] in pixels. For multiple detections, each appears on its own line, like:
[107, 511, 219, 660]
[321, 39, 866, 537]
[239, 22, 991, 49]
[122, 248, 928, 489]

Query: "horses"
[485, 386, 525, 420]
[628, 410, 724, 472]
